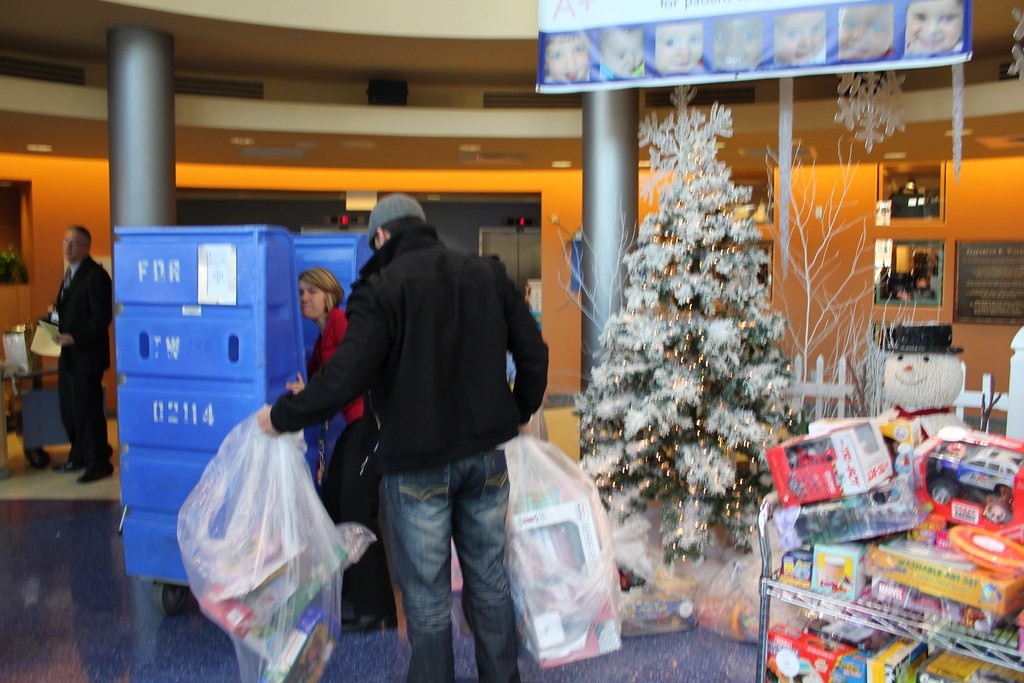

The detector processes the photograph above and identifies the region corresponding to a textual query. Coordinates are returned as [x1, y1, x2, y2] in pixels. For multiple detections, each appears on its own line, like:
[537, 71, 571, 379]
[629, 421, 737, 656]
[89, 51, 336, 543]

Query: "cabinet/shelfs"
[753, 483, 1024, 682]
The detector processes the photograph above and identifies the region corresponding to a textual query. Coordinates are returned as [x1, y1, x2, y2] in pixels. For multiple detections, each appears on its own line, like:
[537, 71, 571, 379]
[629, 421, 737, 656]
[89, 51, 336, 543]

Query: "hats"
[367, 194, 427, 246]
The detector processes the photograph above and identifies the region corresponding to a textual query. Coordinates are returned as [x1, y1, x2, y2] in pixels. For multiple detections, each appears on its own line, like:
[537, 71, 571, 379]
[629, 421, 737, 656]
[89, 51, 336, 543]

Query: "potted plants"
[0, 247, 29, 287]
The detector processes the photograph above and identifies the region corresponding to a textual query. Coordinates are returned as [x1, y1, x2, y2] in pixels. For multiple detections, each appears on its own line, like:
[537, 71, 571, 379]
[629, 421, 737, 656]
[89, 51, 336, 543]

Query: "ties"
[61, 266, 72, 300]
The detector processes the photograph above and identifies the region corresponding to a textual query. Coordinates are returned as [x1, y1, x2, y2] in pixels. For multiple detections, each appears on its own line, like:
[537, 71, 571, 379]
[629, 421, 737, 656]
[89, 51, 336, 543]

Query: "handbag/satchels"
[176, 401, 378, 683]
[500, 434, 623, 669]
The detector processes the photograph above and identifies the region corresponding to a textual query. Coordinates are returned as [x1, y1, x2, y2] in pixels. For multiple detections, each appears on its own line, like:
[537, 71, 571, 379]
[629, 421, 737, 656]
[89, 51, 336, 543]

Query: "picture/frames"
[709, 165, 776, 227]
[874, 235, 947, 311]
[697, 242, 778, 308]
[875, 162, 951, 231]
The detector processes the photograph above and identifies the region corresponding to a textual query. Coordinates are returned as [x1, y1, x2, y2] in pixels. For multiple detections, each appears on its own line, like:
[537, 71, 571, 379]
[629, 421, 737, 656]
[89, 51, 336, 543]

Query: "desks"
[0, 359, 58, 479]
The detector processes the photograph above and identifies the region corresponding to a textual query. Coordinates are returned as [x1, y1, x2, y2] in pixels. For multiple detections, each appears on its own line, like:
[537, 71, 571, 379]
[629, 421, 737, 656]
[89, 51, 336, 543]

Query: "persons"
[542, 0, 970, 87]
[36, 223, 114, 484]
[285, 266, 405, 635]
[256, 194, 550, 683]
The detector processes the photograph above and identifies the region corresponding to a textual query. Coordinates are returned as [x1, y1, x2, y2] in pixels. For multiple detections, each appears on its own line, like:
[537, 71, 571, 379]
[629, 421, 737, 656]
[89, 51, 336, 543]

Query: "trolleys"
[10, 371, 113, 469]
[756, 496, 1024, 682]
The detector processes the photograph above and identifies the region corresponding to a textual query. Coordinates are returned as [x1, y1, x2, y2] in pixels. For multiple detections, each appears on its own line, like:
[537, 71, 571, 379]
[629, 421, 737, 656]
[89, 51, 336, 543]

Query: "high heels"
[339, 604, 398, 631]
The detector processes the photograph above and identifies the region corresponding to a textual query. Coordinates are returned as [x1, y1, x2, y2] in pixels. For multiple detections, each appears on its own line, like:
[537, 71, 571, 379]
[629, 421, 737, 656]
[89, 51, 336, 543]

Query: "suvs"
[927, 447, 1022, 507]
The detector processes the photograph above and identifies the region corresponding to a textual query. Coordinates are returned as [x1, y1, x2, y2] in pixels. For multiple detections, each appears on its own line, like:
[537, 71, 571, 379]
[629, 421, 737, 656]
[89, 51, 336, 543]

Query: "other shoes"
[51, 458, 113, 482]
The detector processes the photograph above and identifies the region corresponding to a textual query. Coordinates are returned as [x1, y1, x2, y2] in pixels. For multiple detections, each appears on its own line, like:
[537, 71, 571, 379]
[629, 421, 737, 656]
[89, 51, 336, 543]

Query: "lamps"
[364, 71, 416, 112]
